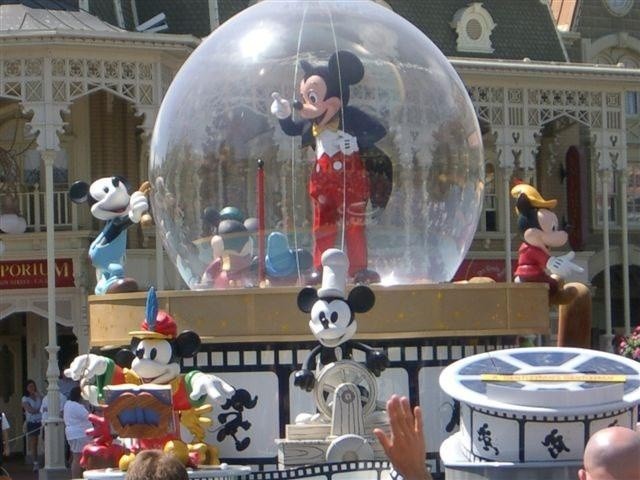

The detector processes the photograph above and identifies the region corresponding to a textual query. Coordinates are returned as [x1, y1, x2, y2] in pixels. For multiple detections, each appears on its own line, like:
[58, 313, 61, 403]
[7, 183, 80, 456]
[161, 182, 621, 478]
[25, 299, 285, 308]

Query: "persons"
[610, 335, 627, 354]
[578, 426, 639, 480]
[0, 379, 97, 479]
[373, 393, 431, 480]
[124, 449, 188, 479]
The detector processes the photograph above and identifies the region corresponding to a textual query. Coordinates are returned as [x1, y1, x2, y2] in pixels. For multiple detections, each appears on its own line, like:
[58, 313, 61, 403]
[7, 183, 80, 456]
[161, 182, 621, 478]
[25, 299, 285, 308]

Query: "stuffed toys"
[201, 206, 260, 288]
[512, 184, 585, 305]
[269, 50, 391, 285]
[63, 287, 235, 470]
[294, 247, 391, 424]
[67, 173, 148, 296]
[251, 230, 311, 285]
